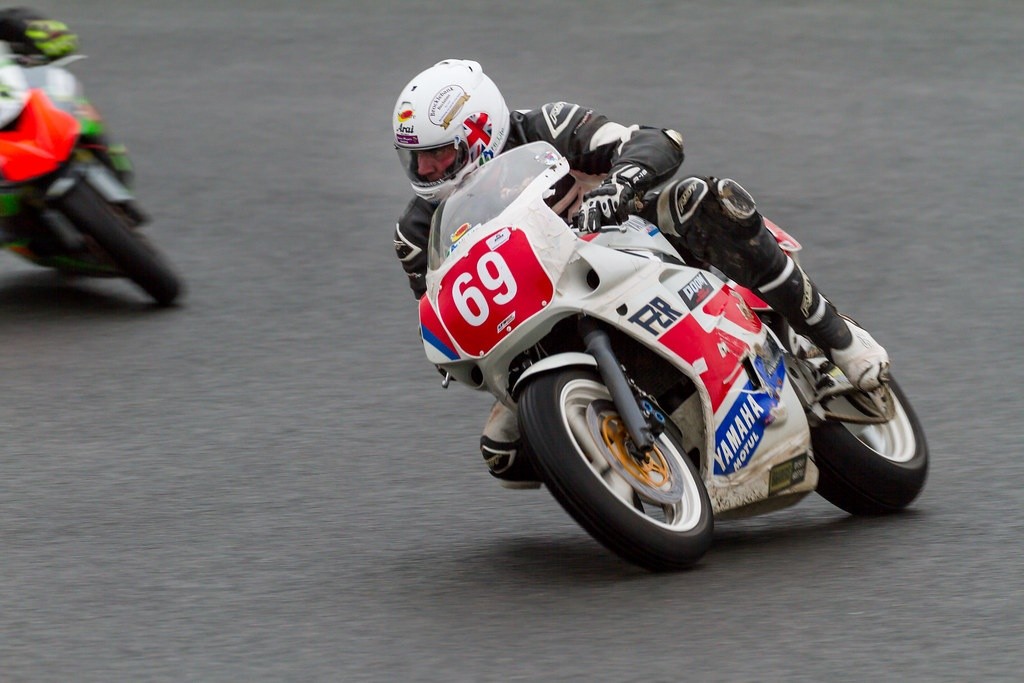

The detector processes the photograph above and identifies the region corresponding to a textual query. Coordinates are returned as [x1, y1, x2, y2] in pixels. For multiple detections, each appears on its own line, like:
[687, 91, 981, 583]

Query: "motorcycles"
[3, 50, 186, 304]
[418, 141, 931, 575]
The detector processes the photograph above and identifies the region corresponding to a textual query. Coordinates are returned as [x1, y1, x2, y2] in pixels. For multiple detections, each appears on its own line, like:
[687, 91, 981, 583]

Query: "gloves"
[576, 162, 651, 235]
[23, 18, 78, 60]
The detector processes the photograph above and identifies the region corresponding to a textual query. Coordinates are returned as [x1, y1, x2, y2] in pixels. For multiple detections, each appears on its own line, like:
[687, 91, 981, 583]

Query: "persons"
[391, 59, 891, 490]
[1, 2, 152, 274]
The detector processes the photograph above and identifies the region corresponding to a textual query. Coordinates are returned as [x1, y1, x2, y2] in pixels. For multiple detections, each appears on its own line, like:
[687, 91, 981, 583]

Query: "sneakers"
[824, 313, 890, 390]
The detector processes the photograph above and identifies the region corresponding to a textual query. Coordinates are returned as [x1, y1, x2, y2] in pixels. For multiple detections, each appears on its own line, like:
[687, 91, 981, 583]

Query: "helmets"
[392, 59, 510, 205]
